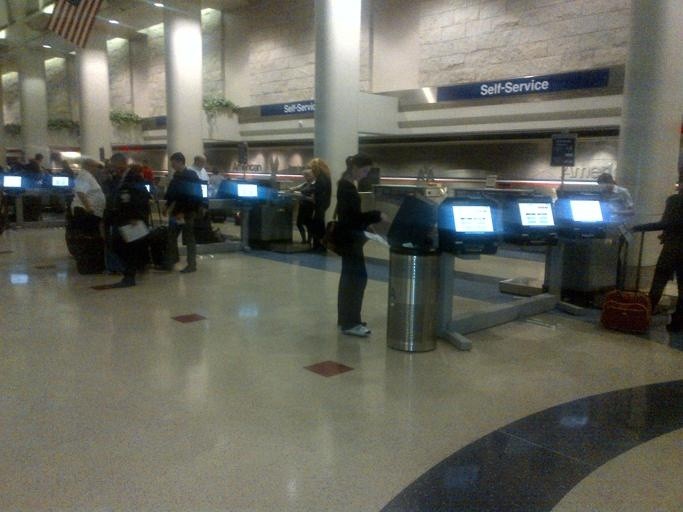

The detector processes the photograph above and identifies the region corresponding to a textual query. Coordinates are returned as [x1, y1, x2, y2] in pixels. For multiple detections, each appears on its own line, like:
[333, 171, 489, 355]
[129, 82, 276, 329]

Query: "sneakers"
[75, 265, 197, 289]
[339, 319, 373, 338]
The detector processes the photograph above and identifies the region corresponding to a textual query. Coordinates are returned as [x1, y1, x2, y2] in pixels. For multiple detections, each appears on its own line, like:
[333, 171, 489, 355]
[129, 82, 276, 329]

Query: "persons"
[624, 181, 682, 334]
[189, 155, 209, 181]
[69, 156, 105, 274]
[306, 157, 332, 254]
[595, 173, 634, 215]
[284, 168, 314, 246]
[158, 152, 202, 273]
[107, 153, 149, 288]
[208, 168, 224, 190]
[334, 152, 392, 336]
[0, 146, 161, 235]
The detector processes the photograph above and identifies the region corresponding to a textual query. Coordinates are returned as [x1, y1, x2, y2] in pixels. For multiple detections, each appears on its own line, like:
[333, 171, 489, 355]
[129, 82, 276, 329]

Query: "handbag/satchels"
[322, 221, 353, 255]
[186, 213, 212, 234]
[600, 288, 650, 334]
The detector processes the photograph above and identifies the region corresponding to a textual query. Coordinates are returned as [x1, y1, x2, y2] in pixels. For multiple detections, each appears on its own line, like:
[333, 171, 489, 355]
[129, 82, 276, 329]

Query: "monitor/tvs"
[438, 191, 608, 242]
[0, 175, 73, 192]
[142, 183, 258, 201]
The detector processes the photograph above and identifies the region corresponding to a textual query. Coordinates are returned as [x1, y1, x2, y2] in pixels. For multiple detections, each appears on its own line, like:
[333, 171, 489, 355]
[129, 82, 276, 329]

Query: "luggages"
[78, 234, 104, 271]
[150, 225, 179, 267]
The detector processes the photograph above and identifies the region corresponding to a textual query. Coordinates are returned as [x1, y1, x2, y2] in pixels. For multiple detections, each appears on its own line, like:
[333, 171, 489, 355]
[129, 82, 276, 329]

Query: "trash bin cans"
[386, 247, 441, 352]
